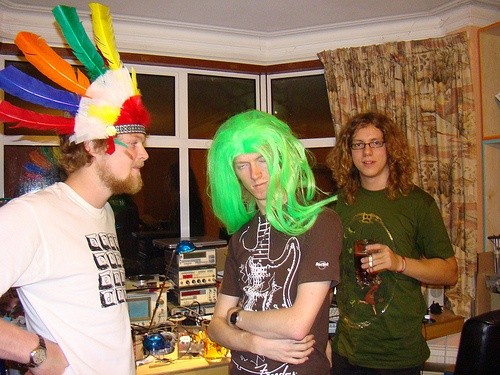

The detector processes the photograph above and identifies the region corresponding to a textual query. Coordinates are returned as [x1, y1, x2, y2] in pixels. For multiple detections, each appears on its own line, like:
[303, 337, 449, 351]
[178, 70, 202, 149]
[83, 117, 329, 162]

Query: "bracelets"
[398, 254, 407, 275]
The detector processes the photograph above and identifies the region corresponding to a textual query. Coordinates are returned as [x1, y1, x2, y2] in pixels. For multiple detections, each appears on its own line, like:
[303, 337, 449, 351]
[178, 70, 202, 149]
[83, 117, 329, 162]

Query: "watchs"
[229, 307, 243, 328]
[18, 335, 47, 372]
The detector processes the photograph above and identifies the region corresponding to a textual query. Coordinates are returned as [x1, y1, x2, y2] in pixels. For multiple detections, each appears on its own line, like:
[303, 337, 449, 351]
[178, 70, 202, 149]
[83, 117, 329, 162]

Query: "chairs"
[445, 309, 500, 375]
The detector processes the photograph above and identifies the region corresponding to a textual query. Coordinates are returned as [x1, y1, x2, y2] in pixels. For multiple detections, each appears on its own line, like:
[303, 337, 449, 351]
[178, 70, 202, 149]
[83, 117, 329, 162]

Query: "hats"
[0, 0, 152, 144]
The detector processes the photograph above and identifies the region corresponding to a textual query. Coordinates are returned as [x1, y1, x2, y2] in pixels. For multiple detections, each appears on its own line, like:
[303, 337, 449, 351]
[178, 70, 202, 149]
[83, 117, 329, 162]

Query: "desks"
[138, 340, 231, 375]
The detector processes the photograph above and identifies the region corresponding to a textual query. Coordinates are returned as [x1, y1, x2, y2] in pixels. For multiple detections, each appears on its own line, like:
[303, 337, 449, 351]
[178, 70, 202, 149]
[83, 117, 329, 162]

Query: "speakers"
[424, 284, 444, 309]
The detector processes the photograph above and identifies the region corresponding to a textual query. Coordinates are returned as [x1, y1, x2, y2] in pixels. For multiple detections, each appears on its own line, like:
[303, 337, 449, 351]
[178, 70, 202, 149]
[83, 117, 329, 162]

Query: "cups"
[201, 320, 229, 363]
[354, 238, 377, 286]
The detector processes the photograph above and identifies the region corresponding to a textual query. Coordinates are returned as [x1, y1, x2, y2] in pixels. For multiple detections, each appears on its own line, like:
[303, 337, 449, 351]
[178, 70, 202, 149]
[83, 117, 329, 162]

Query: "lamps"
[144, 241, 197, 354]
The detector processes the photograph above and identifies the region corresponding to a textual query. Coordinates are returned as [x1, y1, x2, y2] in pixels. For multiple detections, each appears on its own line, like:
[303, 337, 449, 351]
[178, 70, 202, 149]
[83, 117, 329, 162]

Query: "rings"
[366, 253, 375, 275]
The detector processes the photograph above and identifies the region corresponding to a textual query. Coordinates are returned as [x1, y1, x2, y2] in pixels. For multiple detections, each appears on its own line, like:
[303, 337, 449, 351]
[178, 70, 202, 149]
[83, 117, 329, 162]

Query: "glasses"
[349, 141, 387, 149]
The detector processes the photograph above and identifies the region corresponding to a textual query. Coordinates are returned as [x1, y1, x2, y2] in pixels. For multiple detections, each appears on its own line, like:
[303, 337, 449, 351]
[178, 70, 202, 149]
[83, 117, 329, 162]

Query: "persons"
[199, 110, 344, 375]
[319, 111, 459, 375]
[0, 1, 149, 375]
